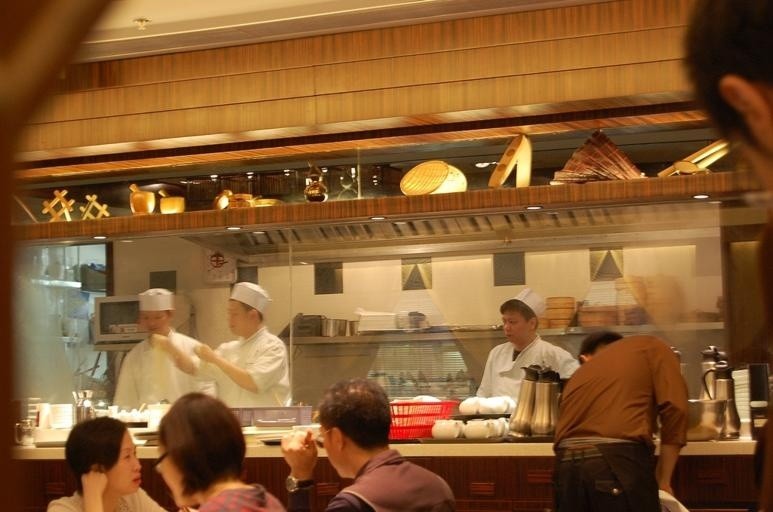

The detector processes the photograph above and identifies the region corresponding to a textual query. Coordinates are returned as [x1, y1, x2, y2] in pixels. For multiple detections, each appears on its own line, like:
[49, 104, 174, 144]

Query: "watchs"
[286, 476, 314, 489]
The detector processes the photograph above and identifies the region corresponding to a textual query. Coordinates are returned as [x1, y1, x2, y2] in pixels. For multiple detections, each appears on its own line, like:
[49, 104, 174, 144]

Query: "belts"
[559, 447, 605, 463]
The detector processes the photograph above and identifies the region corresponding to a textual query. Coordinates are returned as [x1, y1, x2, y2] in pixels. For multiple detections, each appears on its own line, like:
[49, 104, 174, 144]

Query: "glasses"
[150, 451, 172, 478]
[313, 428, 334, 450]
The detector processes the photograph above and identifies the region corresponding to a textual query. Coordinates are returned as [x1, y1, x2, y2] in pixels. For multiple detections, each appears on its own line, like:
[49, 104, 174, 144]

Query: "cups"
[459, 396, 517, 415]
[464, 417, 510, 440]
[431, 419, 465, 440]
[14, 419, 34, 445]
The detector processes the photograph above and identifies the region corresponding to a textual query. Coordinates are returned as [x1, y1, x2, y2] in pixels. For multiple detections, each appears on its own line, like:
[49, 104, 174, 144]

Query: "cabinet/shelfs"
[9, 434, 758, 512]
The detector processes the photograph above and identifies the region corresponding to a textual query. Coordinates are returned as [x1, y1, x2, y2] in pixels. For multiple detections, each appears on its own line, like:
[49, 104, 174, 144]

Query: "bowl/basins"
[320, 316, 348, 337]
[685, 400, 722, 442]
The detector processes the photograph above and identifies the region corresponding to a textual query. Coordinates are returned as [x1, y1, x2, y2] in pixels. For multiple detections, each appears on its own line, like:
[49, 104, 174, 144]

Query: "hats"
[509, 288, 549, 321]
[229, 280, 273, 320]
[138, 289, 177, 313]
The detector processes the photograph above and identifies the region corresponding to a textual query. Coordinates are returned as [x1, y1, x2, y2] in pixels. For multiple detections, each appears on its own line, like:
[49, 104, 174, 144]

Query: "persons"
[47, 417, 168, 512]
[113, 289, 217, 411]
[554, 331, 689, 512]
[684, 0, 773, 314]
[281, 378, 456, 512]
[153, 393, 286, 512]
[476, 299, 580, 405]
[151, 282, 292, 407]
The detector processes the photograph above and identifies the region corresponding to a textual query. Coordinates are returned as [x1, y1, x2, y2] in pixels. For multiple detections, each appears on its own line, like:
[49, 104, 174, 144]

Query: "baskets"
[387, 400, 462, 442]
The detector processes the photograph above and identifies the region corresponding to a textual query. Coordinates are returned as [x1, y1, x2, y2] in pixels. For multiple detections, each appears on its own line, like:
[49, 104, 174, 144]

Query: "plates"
[359, 313, 396, 333]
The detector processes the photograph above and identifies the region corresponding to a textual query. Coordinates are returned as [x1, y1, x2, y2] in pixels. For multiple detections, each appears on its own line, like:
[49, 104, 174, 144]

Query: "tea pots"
[699, 345, 741, 441]
[512, 365, 560, 436]
